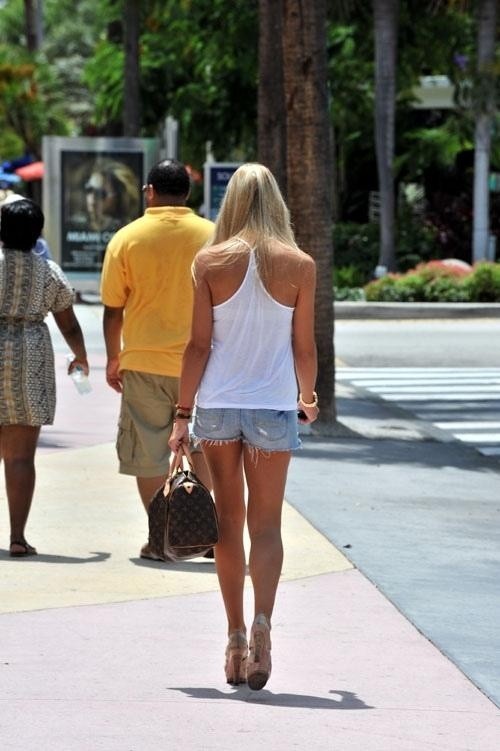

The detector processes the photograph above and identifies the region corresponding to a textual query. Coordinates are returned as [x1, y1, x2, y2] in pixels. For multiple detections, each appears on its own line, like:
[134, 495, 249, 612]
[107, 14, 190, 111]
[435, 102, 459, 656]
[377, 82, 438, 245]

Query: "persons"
[96, 157, 215, 565]
[166, 160, 321, 694]
[69, 156, 141, 230]
[0, 197, 92, 561]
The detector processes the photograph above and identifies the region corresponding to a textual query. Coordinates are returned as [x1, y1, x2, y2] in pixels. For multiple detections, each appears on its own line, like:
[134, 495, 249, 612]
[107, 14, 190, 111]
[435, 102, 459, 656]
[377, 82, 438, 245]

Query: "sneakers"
[139, 541, 162, 562]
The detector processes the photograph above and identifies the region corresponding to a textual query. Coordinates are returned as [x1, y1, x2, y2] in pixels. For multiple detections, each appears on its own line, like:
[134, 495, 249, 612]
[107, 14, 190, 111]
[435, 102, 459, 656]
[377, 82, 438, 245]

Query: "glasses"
[82, 182, 107, 202]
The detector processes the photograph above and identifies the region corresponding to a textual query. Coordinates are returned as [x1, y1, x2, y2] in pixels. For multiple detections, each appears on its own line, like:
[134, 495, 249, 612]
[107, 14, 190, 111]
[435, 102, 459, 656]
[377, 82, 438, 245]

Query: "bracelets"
[299, 390, 318, 409]
[175, 403, 193, 413]
[173, 410, 191, 421]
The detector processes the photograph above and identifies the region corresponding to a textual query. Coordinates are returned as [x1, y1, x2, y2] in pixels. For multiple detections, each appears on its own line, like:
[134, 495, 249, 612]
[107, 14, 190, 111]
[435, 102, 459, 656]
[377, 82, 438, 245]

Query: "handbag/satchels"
[147, 442, 219, 561]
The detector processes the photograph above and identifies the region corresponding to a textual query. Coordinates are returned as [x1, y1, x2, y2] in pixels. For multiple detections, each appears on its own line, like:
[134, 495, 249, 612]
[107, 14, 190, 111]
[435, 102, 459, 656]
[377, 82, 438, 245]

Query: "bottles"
[64, 350, 92, 396]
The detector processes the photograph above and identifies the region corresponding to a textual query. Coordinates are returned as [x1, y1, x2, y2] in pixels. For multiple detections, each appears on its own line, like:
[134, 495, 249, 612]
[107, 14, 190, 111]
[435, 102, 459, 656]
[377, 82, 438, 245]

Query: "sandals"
[10, 540, 36, 556]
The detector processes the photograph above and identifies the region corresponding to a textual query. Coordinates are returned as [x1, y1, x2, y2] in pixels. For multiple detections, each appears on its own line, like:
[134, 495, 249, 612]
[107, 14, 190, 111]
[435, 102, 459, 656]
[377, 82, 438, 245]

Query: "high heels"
[247, 615, 271, 691]
[225, 629, 248, 687]
